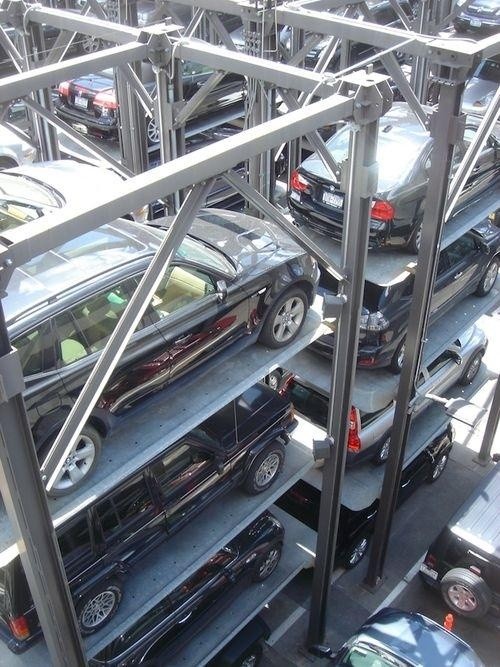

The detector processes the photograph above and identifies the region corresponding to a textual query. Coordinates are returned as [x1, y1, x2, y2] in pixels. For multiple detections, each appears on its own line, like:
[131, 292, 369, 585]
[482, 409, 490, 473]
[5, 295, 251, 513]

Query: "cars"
[221, 16, 291, 56]
[0, 7, 102, 77]
[54, 57, 248, 147]
[458, 55, 500, 120]
[0, 117, 37, 170]
[330, 607, 484, 666]
[88, 511, 285, 667]
[282, 423, 453, 569]
[453, 0, 500, 33]
[0, 160, 124, 230]
[285, 100, 500, 256]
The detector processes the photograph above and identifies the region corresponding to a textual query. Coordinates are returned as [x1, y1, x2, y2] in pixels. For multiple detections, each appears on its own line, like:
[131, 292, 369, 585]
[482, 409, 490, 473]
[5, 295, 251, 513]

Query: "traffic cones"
[444, 613, 454, 632]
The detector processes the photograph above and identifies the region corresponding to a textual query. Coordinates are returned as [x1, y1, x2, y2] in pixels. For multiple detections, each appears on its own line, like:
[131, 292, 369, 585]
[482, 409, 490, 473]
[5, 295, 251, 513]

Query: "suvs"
[305, 217, 500, 374]
[418, 461, 500, 631]
[0, 382, 299, 655]
[279, 322, 489, 466]
[220, 616, 271, 666]
[282, 0, 413, 68]
[0, 208, 321, 496]
